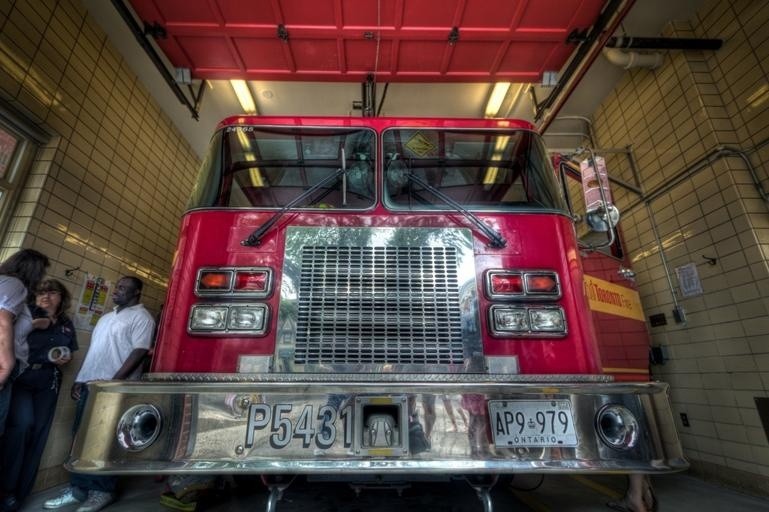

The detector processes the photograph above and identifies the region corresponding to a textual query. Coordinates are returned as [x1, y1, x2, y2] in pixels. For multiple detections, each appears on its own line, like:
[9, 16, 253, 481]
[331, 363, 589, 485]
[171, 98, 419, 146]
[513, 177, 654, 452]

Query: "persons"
[43, 277, 156, 512]
[0, 249, 79, 512]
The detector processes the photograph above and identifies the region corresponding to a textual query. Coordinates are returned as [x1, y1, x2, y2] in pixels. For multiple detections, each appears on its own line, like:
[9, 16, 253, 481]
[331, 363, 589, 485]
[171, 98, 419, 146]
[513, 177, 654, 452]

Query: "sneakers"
[43, 488, 116, 512]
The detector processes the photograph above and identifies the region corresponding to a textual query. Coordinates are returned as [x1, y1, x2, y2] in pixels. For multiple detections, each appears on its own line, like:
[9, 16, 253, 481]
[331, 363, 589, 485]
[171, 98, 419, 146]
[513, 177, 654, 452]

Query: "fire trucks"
[62, 115, 690, 512]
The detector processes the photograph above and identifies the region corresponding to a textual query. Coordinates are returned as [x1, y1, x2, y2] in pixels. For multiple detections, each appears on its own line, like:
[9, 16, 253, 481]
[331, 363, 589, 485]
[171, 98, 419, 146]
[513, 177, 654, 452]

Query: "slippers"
[607, 474, 658, 512]
